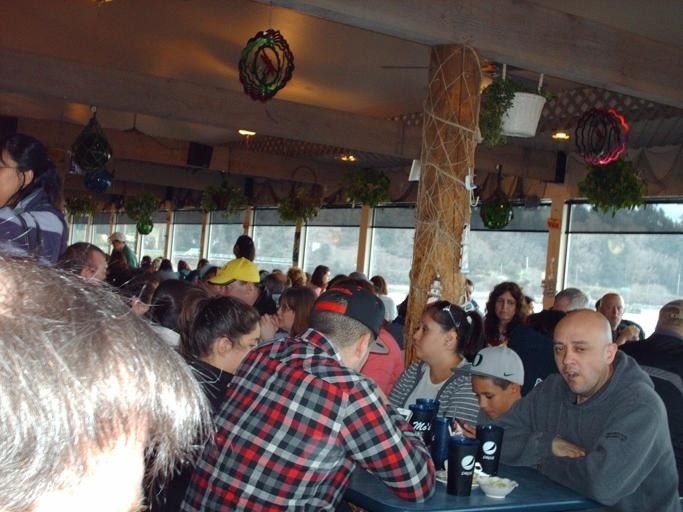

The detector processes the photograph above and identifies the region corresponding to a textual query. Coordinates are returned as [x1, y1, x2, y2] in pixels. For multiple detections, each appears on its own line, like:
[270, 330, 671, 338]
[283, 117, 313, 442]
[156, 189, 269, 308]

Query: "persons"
[1, 132, 682, 512]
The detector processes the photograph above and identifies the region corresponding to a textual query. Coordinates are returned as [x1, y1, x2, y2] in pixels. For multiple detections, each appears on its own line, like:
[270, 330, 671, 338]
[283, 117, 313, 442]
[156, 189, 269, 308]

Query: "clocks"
[237, 28, 295, 102]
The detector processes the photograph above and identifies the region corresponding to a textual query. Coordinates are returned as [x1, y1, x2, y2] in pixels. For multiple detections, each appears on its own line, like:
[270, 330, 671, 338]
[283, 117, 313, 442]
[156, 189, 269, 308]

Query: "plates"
[433, 470, 489, 490]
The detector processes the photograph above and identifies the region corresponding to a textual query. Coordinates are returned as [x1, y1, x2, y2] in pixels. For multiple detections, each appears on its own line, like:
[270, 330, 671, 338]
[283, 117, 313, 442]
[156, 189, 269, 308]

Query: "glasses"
[434, 298, 463, 336]
[275, 302, 293, 313]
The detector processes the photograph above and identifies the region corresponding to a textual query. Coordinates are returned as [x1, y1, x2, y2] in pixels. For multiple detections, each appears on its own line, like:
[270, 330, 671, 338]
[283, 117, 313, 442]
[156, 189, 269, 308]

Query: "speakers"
[187, 142, 213, 168]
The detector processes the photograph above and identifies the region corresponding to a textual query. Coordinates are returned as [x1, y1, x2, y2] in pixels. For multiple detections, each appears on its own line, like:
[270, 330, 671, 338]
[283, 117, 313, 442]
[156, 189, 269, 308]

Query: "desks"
[344, 465, 600, 512]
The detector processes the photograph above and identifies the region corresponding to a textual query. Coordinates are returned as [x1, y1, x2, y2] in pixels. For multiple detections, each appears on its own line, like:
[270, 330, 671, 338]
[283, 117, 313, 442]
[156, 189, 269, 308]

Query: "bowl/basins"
[477, 476, 519, 498]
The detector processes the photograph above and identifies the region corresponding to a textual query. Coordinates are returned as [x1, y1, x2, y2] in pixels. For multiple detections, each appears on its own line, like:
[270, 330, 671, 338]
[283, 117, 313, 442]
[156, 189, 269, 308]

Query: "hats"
[449, 346, 525, 387]
[206, 258, 261, 285]
[310, 283, 391, 359]
[108, 231, 128, 243]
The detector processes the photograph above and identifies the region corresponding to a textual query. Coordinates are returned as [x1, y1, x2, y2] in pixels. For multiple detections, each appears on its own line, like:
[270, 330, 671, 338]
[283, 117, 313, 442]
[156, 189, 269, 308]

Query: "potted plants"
[276, 162, 324, 226]
[479, 64, 557, 150]
[578, 151, 651, 218]
[199, 179, 248, 221]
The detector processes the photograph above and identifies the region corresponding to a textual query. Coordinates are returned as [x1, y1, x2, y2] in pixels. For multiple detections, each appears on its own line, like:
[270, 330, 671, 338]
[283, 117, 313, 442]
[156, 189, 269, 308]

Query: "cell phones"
[396, 406, 414, 423]
[443, 416, 464, 428]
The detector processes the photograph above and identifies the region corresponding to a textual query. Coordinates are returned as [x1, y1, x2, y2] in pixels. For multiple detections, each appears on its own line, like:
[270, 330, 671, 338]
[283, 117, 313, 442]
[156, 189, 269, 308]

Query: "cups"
[408, 398, 458, 471]
[447, 435, 479, 497]
[475, 424, 503, 476]
